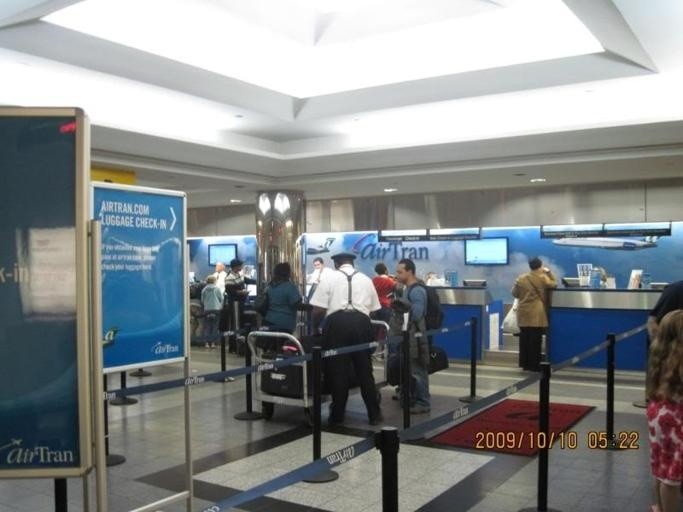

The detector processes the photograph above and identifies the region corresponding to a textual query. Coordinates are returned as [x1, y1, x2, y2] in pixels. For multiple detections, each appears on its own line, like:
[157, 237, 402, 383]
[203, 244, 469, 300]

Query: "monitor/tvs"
[207, 243, 237, 267]
[650, 282, 670, 290]
[463, 279, 487, 287]
[562, 278, 579, 286]
[465, 236, 509, 266]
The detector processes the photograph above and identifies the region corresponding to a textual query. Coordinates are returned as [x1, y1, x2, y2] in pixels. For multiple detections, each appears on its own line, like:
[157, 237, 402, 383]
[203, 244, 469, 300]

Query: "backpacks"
[406, 281, 444, 330]
[189, 282, 207, 301]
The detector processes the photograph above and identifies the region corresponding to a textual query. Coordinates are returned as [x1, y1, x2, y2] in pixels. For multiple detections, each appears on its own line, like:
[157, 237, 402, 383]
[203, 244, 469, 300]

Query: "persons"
[645, 309, 683, 511]
[511, 256, 558, 372]
[645, 280, 683, 349]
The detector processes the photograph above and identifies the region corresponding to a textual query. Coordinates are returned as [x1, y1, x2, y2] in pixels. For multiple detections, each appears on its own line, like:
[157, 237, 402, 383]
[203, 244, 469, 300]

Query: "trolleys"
[239, 313, 393, 422]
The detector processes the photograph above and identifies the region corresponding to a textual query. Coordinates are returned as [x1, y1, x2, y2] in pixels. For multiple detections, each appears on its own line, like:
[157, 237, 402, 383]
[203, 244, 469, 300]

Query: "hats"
[205, 274, 218, 283]
[231, 259, 243, 268]
[331, 253, 356, 259]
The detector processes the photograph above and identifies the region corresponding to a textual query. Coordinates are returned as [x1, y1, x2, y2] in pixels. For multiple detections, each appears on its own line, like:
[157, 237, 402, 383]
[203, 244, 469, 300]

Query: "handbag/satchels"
[387, 347, 449, 386]
[255, 291, 269, 315]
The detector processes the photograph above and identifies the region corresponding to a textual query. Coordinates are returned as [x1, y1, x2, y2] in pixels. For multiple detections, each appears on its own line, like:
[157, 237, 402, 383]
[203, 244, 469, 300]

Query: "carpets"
[424, 397, 596, 457]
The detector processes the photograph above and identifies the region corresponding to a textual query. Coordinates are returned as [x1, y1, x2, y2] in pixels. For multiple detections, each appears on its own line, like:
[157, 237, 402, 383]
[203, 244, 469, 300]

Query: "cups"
[640, 273, 651, 291]
[444, 269, 458, 288]
[576, 262, 593, 286]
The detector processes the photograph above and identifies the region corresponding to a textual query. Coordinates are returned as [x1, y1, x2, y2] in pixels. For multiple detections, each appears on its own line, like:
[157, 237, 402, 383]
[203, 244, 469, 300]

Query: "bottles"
[590, 269, 603, 289]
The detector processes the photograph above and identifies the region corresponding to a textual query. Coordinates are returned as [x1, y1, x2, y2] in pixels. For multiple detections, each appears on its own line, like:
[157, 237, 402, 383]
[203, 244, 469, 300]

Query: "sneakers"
[391, 388, 403, 401]
[191, 340, 216, 348]
[327, 419, 345, 430]
[369, 411, 384, 425]
[409, 401, 431, 414]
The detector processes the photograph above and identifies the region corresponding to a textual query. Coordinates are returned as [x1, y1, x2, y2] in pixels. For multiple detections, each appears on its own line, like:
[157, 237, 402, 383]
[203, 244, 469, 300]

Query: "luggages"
[259, 350, 315, 400]
[295, 333, 363, 395]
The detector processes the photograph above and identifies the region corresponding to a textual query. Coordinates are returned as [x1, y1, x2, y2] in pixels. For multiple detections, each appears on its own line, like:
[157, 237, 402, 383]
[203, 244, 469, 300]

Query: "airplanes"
[306, 237, 334, 254]
[551, 234, 661, 251]
